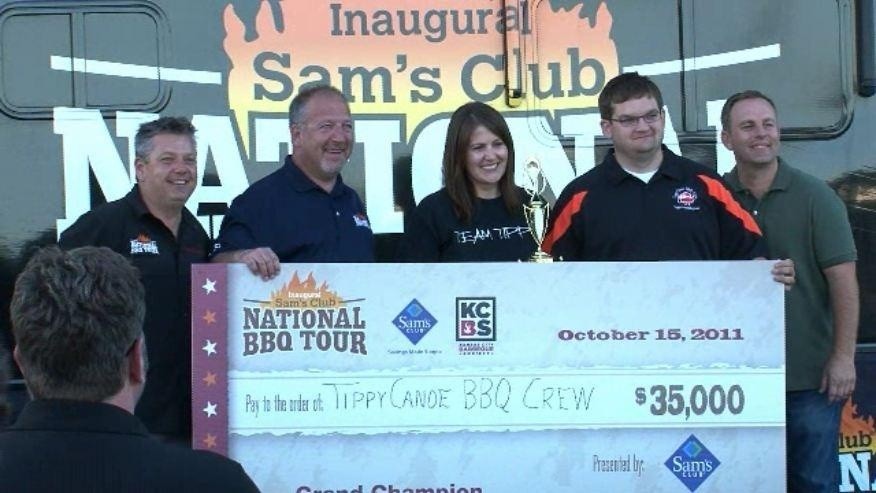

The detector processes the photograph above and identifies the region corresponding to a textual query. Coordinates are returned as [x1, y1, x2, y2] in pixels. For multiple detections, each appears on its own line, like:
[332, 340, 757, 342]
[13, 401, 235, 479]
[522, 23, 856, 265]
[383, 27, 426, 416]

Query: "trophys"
[523, 155, 553, 262]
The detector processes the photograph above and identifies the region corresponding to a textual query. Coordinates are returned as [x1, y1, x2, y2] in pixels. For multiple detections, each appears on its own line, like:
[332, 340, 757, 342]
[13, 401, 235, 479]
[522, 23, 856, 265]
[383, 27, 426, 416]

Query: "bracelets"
[238, 248, 252, 256]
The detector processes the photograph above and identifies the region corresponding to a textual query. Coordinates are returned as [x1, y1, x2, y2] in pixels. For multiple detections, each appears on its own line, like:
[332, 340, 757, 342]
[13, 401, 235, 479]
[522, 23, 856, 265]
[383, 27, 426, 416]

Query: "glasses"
[608, 109, 661, 127]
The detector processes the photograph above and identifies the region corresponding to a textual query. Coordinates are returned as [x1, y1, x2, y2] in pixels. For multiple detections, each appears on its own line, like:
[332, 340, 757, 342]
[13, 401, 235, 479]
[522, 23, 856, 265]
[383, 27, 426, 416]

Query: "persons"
[208, 86, 375, 281]
[721, 88, 862, 492]
[56, 112, 212, 448]
[402, 101, 552, 262]
[0, 243, 261, 493]
[542, 72, 796, 293]
